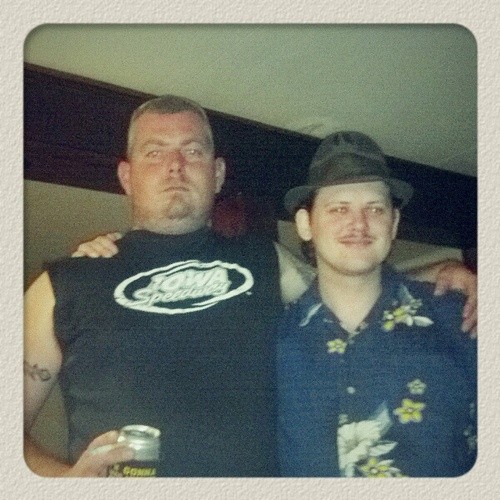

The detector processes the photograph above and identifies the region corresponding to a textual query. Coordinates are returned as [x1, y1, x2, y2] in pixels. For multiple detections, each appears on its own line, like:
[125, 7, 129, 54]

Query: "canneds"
[107, 424, 162, 476]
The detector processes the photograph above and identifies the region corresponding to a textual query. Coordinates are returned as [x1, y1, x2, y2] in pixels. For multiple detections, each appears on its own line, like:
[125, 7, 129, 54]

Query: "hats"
[283, 130, 413, 210]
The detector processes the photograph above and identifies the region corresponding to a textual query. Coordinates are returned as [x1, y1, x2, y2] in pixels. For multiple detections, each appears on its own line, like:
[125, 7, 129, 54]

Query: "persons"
[73, 130, 477, 477]
[24, 93, 479, 477]
